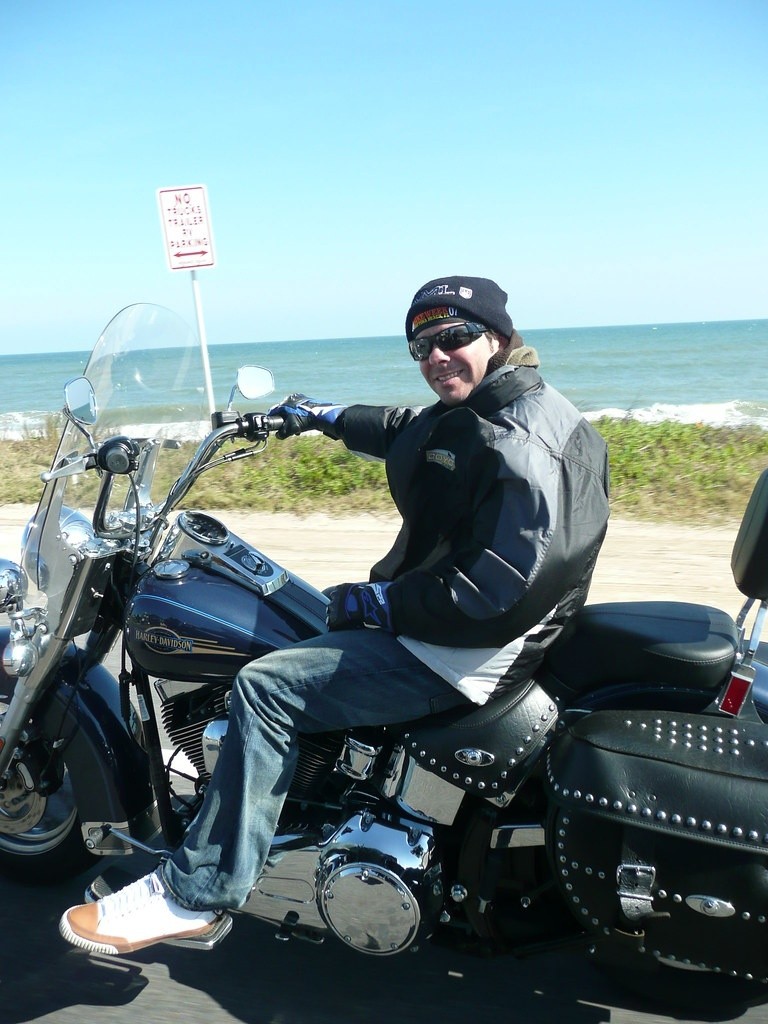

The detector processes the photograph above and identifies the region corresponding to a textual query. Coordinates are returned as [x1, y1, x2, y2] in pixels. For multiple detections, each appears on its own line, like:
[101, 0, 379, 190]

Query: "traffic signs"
[160, 185, 214, 269]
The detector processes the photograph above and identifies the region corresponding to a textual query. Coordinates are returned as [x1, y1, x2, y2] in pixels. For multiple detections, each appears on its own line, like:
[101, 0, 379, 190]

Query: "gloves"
[322, 581, 398, 632]
[267, 393, 348, 441]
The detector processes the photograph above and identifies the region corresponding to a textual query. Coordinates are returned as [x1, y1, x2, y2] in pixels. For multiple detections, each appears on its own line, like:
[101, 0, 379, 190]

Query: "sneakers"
[59, 869, 223, 955]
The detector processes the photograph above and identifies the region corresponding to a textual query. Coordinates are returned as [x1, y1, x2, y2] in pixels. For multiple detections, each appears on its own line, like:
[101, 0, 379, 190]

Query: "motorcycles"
[0, 304, 768, 1022]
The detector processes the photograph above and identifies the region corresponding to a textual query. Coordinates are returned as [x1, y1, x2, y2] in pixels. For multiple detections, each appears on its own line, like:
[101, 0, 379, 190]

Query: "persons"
[59, 276, 608, 955]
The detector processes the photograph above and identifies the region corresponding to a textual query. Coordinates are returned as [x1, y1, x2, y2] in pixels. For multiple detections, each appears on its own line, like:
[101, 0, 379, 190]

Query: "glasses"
[408, 320, 489, 361]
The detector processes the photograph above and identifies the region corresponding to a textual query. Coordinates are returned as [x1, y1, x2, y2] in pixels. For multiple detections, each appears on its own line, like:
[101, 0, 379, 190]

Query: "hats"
[405, 275, 512, 342]
